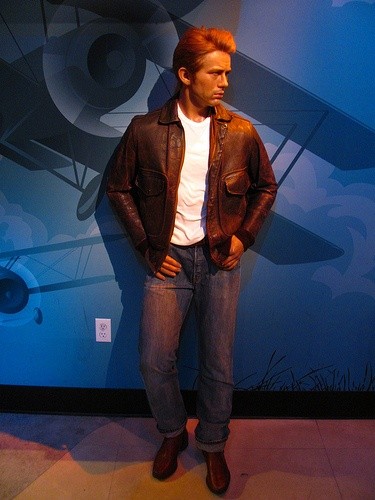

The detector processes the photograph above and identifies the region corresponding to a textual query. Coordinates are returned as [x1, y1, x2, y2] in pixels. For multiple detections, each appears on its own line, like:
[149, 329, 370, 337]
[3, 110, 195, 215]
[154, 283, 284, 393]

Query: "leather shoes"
[206, 452, 230, 495]
[153, 428, 188, 479]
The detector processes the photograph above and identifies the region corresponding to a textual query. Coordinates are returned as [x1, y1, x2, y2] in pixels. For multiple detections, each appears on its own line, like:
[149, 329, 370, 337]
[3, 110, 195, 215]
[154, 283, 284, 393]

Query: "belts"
[190, 238, 208, 248]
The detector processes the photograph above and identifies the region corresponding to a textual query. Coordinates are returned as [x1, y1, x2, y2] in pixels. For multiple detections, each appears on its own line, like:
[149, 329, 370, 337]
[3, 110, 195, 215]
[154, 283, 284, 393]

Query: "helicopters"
[0, 232, 138, 325]
[0, 0, 375, 265]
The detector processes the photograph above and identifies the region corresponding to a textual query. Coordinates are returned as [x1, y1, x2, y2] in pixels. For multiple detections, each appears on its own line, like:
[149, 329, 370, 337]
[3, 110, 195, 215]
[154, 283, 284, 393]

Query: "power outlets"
[95, 318, 111, 342]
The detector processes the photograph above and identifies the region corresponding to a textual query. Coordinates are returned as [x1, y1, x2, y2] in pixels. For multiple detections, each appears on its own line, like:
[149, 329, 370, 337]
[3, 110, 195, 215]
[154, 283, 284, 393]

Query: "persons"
[103, 25, 278, 496]
